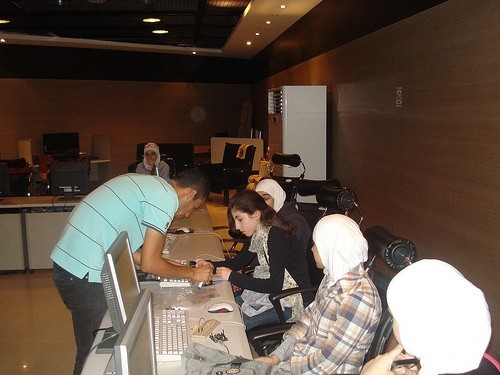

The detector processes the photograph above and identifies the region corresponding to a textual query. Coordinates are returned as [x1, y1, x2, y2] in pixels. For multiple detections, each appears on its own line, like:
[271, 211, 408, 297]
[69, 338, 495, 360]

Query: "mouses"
[175, 227, 190, 234]
[207, 302, 234, 313]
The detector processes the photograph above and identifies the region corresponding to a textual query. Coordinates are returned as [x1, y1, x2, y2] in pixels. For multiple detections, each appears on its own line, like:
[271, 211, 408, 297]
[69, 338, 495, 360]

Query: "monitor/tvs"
[43, 132, 80, 160]
[0, 163, 8, 201]
[103, 288, 158, 375]
[95, 231, 142, 354]
[49, 160, 88, 202]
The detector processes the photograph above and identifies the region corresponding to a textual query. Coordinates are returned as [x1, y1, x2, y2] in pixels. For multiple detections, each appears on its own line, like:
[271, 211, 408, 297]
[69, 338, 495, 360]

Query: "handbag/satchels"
[181, 344, 272, 375]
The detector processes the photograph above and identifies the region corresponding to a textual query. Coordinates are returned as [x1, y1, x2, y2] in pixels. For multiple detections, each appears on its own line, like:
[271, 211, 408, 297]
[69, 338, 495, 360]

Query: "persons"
[253, 213, 382, 375]
[228, 179, 311, 296]
[193, 189, 324, 356]
[49, 166, 214, 375]
[135, 142, 170, 182]
[358, 258, 499, 375]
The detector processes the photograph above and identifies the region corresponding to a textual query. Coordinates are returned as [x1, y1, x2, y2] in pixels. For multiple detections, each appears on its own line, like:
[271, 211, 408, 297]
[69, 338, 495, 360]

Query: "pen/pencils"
[392, 358, 419, 365]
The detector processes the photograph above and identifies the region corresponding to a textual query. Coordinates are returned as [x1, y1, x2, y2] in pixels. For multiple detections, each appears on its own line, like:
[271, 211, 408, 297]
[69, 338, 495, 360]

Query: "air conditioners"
[267, 86, 327, 203]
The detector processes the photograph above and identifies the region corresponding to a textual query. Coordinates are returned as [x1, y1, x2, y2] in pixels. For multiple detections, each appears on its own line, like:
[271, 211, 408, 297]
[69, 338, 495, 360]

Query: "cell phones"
[390, 359, 420, 372]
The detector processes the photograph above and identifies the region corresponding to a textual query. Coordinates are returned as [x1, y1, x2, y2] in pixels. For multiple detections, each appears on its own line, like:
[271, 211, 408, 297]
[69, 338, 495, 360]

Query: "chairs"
[0, 128, 500, 375]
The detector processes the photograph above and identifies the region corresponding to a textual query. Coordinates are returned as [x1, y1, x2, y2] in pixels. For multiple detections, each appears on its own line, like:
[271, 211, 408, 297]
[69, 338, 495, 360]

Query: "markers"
[195, 280, 206, 290]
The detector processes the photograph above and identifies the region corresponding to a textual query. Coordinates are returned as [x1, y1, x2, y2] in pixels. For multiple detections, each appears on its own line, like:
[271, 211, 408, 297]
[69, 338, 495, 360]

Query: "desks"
[31, 160, 111, 193]
[81, 232, 253, 375]
[0, 195, 86, 275]
[169, 201, 214, 233]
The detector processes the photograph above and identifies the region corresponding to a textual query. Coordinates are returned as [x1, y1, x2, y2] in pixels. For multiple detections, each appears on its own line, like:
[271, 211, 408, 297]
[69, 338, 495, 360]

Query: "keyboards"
[158, 259, 192, 287]
[152, 309, 189, 362]
[161, 232, 180, 254]
